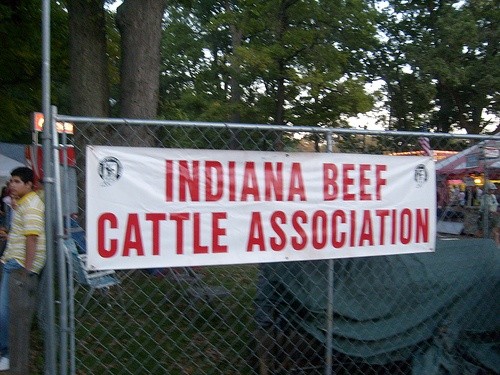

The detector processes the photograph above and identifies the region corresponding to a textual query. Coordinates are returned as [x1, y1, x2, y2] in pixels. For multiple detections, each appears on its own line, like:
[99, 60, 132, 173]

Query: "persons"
[445, 184, 482, 208]
[476, 182, 500, 245]
[0, 167, 46, 375]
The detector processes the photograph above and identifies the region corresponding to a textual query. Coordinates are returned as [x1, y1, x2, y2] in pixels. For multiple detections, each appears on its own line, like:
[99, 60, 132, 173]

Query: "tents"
[435, 132, 500, 181]
